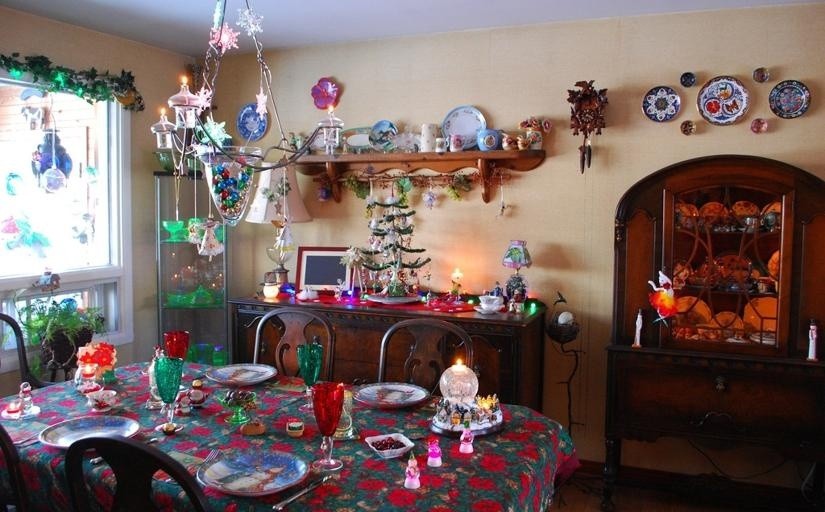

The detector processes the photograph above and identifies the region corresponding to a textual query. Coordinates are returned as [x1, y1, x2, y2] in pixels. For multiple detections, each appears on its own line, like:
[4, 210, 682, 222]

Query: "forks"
[165, 448, 220, 484]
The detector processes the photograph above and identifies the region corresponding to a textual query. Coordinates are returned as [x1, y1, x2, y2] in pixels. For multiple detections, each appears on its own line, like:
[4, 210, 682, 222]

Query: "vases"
[527, 130, 544, 151]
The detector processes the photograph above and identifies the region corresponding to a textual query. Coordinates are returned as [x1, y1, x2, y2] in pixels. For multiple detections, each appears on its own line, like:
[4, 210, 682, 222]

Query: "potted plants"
[14, 288, 107, 382]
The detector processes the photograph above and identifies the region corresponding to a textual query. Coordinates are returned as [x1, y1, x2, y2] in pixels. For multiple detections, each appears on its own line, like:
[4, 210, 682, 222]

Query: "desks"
[46, 364, 78, 382]
[226, 282, 548, 417]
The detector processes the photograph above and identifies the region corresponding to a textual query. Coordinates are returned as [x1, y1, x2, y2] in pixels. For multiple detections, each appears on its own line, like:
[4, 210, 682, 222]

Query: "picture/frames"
[294, 246, 356, 295]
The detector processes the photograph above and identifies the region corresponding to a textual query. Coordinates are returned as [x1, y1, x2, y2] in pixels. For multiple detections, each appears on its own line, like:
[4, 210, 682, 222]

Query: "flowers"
[518, 113, 555, 135]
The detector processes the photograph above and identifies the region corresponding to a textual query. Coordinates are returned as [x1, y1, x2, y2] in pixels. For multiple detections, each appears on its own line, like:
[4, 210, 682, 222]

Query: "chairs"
[0, 312, 44, 392]
[0, 424, 34, 512]
[376, 317, 474, 398]
[251, 307, 336, 383]
[65, 433, 213, 512]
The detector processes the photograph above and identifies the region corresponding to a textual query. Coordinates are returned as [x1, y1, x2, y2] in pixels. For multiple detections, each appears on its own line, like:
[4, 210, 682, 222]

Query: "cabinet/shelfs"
[152, 168, 229, 365]
[595, 153, 824, 511]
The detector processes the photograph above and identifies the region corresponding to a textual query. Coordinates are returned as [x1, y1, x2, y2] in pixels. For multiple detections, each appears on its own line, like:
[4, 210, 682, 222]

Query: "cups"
[479, 295, 503, 310]
[175, 106, 195, 128]
[476, 129, 533, 151]
[421, 122, 467, 153]
[323, 127, 339, 149]
[335, 390, 354, 437]
[156, 132, 172, 149]
[4, 396, 23, 415]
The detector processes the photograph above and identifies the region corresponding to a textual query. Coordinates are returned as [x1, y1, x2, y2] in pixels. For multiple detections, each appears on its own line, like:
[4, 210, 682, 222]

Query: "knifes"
[271, 474, 338, 509]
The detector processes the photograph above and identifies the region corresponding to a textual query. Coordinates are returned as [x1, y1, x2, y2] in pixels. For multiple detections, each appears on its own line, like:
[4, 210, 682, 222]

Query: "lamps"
[149, 0, 348, 272]
[261, 271, 280, 298]
[501, 240, 532, 300]
[245, 162, 312, 283]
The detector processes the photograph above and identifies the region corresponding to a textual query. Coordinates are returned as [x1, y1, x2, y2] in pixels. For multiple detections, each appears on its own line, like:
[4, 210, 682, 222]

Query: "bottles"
[286, 130, 310, 155]
[632, 309, 645, 349]
[806, 317, 818, 361]
[186, 379, 208, 405]
[319, 110, 346, 128]
[167, 84, 201, 106]
[187, 342, 225, 365]
[72, 363, 105, 395]
[150, 116, 175, 133]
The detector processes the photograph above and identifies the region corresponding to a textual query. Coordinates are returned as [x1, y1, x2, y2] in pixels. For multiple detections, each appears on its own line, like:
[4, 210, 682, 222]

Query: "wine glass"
[310, 380, 344, 472]
[215, 392, 256, 424]
[147, 330, 195, 434]
[295, 342, 324, 413]
[162, 217, 224, 242]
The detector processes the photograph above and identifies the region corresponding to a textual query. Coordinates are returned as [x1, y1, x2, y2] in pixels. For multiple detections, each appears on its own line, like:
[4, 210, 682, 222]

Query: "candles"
[449, 359, 468, 373]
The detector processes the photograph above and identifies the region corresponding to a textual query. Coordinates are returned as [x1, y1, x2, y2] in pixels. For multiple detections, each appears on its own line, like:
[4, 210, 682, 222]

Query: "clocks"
[565, 77, 608, 173]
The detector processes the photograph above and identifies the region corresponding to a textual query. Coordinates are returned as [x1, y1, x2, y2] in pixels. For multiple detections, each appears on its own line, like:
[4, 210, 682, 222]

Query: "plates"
[203, 363, 279, 388]
[350, 381, 431, 409]
[474, 304, 505, 314]
[339, 120, 399, 153]
[37, 414, 140, 450]
[671, 197, 783, 348]
[642, 86, 682, 123]
[196, 448, 310, 497]
[768, 80, 813, 119]
[441, 106, 486, 150]
[698, 76, 752, 128]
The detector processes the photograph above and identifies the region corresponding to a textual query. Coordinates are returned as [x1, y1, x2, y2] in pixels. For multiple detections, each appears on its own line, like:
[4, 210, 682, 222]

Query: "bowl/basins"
[680, 71, 696, 87]
[365, 432, 415, 459]
[750, 118, 770, 134]
[753, 67, 771, 83]
[85, 389, 116, 413]
[680, 120, 698, 135]
